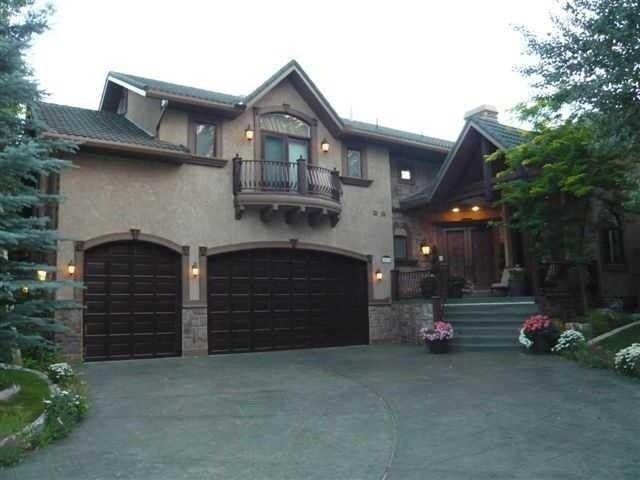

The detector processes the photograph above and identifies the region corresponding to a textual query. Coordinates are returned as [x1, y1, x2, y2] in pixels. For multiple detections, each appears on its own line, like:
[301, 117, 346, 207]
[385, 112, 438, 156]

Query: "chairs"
[490, 268, 511, 297]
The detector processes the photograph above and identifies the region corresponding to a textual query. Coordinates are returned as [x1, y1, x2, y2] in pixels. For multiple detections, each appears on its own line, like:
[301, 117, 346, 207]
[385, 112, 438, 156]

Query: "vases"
[426, 339, 448, 354]
[534, 334, 550, 354]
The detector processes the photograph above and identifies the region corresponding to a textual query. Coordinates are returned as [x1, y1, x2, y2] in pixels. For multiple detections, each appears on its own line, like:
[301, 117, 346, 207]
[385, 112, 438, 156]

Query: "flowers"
[523, 313, 551, 336]
[420, 321, 454, 344]
